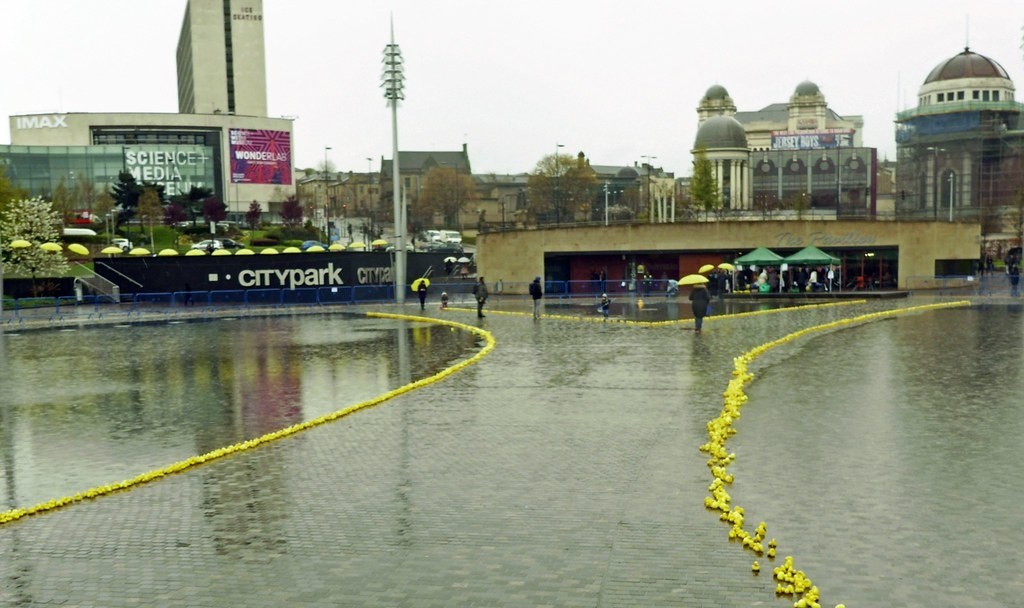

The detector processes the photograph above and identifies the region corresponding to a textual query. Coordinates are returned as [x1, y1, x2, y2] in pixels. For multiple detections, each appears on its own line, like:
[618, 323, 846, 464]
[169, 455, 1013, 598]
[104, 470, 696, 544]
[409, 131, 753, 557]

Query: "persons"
[591, 270, 607, 293]
[601, 294, 610, 317]
[418, 281, 427, 310]
[643, 271, 653, 293]
[707, 266, 839, 296]
[373, 244, 385, 253]
[689, 282, 709, 333]
[446, 259, 453, 275]
[475, 277, 489, 318]
[461, 263, 468, 280]
[530, 276, 543, 319]
[977, 247, 1020, 296]
[441, 291, 448, 307]
[411, 237, 415, 251]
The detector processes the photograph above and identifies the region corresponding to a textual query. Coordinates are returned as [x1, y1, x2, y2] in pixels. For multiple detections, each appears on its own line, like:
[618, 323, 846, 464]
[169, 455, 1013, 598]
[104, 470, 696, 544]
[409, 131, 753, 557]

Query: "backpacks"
[529, 283, 537, 294]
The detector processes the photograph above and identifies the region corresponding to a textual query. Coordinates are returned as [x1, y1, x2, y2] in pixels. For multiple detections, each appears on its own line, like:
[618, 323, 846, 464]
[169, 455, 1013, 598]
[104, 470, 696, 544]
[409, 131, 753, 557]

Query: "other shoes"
[478, 315, 485, 317]
[695, 329, 700, 334]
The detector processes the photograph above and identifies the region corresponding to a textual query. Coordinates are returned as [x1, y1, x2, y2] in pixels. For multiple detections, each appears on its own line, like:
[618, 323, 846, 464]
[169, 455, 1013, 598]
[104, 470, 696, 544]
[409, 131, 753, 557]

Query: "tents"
[783, 244, 840, 293]
[733, 247, 784, 293]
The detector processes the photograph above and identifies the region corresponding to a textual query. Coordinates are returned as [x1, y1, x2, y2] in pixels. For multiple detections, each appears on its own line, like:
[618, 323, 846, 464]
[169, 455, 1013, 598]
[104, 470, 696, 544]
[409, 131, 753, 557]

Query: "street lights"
[556, 144, 566, 223]
[106, 214, 111, 246]
[642, 155, 657, 221]
[110, 210, 116, 237]
[325, 146, 332, 250]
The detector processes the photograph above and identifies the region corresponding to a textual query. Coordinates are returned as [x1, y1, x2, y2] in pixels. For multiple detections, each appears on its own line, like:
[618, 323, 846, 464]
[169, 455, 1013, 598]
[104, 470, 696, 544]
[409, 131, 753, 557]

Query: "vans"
[441, 231, 463, 245]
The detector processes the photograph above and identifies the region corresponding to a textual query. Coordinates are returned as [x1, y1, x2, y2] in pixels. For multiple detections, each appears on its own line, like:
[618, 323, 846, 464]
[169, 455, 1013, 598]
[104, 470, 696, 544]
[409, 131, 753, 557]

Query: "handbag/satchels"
[706, 304, 712, 315]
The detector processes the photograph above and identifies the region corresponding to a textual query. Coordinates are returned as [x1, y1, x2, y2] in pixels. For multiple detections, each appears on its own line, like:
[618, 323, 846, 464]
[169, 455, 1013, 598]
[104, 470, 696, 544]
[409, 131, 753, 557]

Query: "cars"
[112, 238, 134, 251]
[428, 229, 441, 241]
[300, 239, 331, 251]
[129, 233, 152, 247]
[192, 239, 220, 250]
[222, 239, 245, 250]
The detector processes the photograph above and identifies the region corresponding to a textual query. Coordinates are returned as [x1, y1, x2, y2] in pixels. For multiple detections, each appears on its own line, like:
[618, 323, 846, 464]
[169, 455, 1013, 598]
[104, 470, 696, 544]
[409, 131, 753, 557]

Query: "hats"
[602, 293, 607, 298]
[536, 276, 540, 280]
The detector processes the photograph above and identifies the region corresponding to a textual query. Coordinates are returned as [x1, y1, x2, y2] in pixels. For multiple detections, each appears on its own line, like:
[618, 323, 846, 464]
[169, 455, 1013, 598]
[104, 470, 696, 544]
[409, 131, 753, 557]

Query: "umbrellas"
[9, 240, 388, 257]
[444, 257, 470, 263]
[698, 264, 714, 273]
[677, 273, 710, 284]
[411, 278, 430, 292]
[718, 263, 734, 271]
